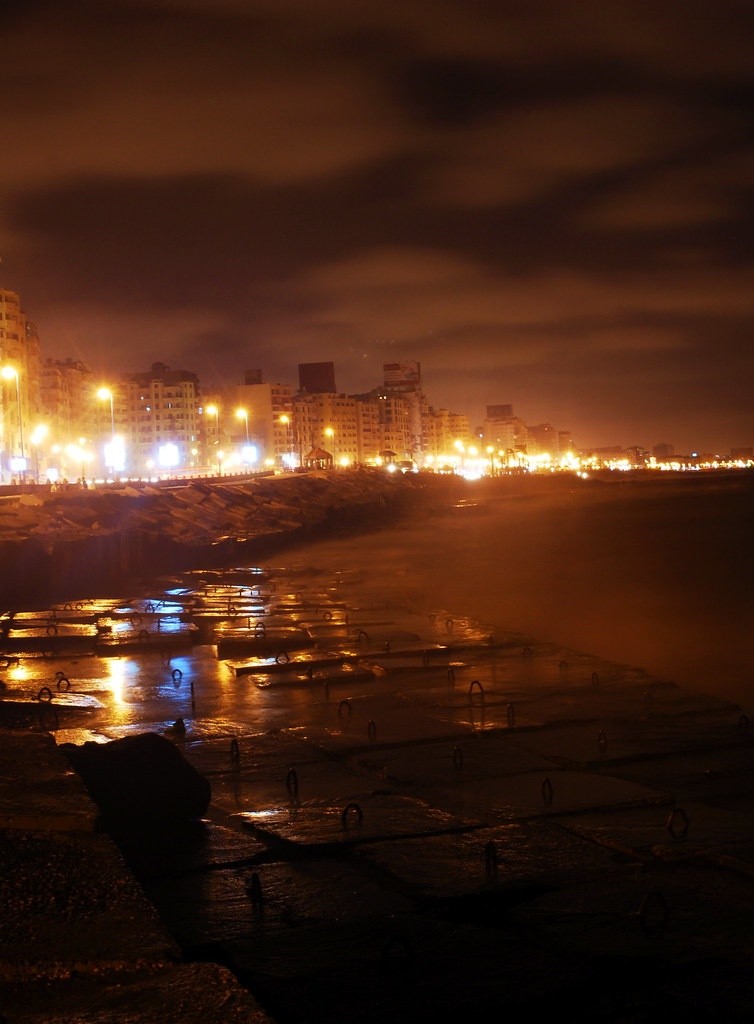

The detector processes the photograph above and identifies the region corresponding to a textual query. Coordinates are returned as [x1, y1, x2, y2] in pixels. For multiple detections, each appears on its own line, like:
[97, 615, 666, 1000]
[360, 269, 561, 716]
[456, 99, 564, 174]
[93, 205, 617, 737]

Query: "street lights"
[278, 414, 294, 473]
[99, 386, 117, 481]
[206, 405, 222, 476]
[236, 408, 252, 472]
[327, 427, 336, 469]
[2, 366, 24, 481]
